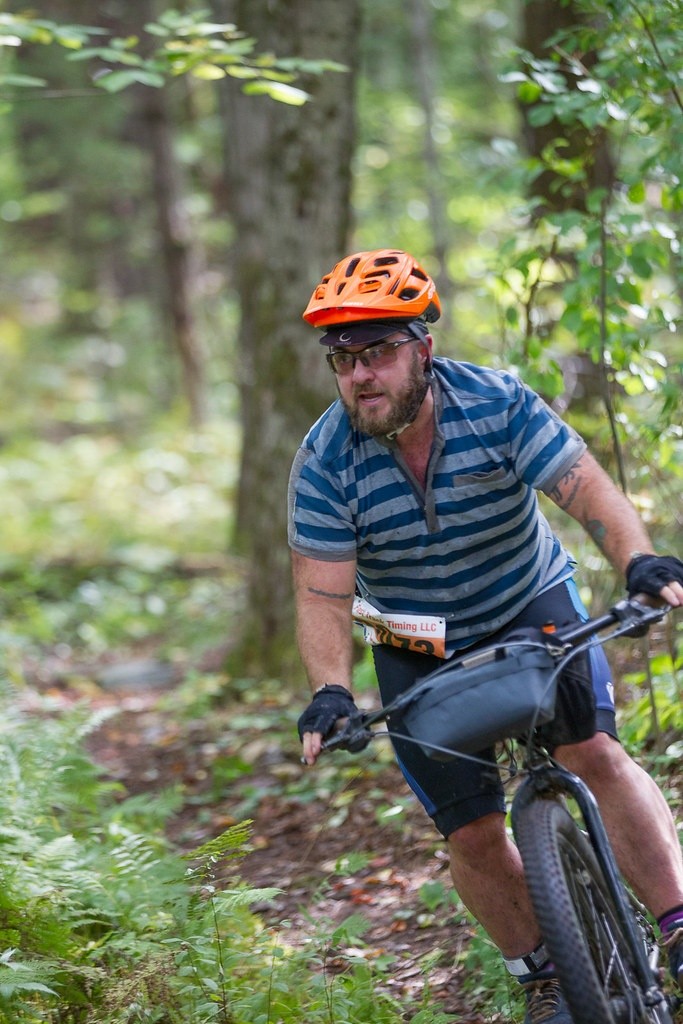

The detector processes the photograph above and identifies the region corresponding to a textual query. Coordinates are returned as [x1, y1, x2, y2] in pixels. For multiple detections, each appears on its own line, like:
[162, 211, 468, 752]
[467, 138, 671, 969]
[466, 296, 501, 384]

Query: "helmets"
[302, 249, 440, 332]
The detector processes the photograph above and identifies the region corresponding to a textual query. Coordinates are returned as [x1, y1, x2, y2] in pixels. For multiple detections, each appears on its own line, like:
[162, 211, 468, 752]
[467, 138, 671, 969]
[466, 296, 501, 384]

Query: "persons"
[289, 250, 683, 1023]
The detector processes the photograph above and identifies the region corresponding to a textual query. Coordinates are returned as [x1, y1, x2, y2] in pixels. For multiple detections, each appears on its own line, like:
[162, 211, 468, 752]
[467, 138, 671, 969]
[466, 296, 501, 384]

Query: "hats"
[319, 318, 429, 346]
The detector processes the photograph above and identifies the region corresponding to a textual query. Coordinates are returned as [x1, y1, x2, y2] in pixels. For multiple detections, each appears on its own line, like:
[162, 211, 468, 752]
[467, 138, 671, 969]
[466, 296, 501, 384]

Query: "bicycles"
[301, 586, 683, 1024]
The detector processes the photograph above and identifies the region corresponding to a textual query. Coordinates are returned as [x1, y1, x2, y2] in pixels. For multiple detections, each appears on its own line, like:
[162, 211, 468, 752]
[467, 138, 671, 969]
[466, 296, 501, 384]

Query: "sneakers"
[657, 919, 683, 988]
[512, 968, 574, 1024]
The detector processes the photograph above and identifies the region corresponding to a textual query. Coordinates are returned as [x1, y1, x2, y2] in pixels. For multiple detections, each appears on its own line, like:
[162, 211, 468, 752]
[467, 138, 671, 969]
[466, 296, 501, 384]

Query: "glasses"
[325, 338, 416, 375]
